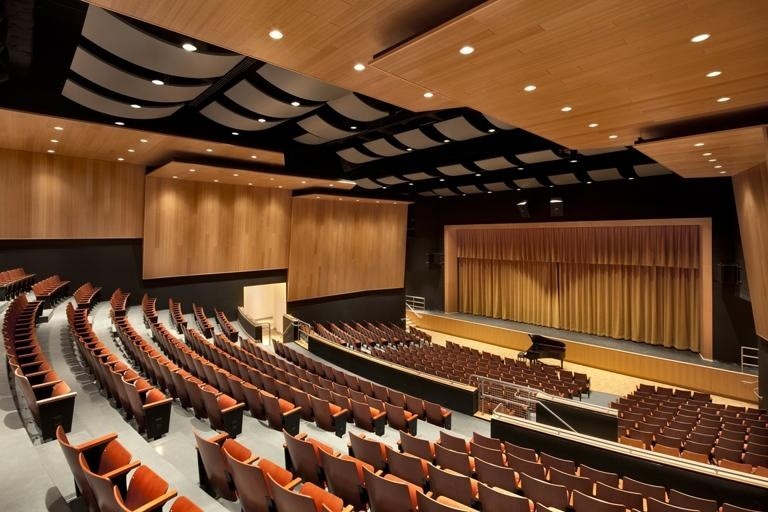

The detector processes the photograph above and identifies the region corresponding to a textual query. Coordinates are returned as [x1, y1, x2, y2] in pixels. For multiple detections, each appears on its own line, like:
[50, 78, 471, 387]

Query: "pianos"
[523, 334, 565, 368]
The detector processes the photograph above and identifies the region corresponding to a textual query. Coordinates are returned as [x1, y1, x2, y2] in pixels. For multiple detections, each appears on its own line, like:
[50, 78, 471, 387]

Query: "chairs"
[0, 268, 234, 317]
[2, 317, 768, 512]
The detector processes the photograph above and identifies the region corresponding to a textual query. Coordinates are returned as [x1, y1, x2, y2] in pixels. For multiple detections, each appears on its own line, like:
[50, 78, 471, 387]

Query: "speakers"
[550, 200, 564, 217]
[424, 253, 440, 265]
[516, 201, 530, 218]
[720, 265, 737, 283]
